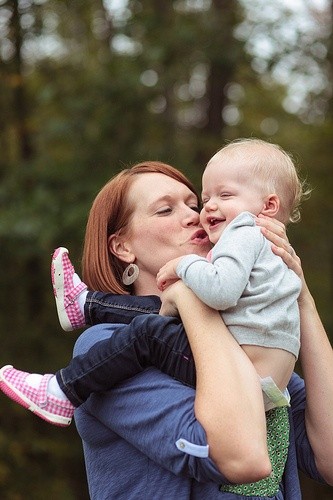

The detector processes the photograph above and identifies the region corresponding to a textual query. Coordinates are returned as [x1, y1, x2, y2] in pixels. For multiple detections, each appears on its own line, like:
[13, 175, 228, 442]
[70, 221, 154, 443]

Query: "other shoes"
[0, 363, 76, 426]
[49, 245, 89, 329]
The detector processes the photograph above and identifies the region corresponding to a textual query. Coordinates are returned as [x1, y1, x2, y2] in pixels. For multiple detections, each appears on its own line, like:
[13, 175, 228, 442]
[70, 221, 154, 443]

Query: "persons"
[0, 135, 308, 435]
[68, 161, 333, 500]
